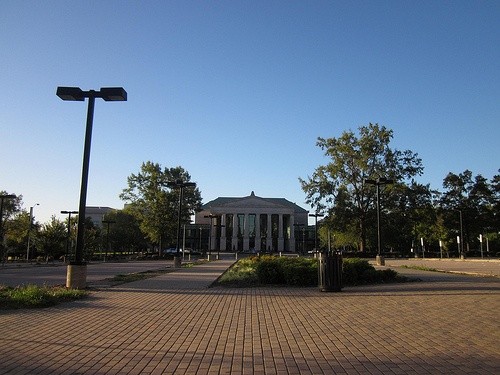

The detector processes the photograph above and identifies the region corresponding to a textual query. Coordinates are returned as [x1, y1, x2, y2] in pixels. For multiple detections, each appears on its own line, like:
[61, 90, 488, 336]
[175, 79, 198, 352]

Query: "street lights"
[364, 177, 394, 266]
[173, 182, 196, 268]
[408, 216, 422, 258]
[204, 215, 221, 262]
[61, 210, 78, 266]
[101, 220, 115, 262]
[27, 203, 39, 260]
[309, 212, 324, 253]
[55, 86, 128, 289]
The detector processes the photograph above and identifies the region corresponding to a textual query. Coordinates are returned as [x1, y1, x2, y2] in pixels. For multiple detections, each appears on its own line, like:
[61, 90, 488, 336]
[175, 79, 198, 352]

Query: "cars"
[308, 247, 324, 254]
[334, 249, 344, 256]
[165, 247, 197, 255]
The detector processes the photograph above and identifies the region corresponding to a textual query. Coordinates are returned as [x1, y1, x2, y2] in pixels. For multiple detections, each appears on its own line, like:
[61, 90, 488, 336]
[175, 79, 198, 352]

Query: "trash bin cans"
[320, 252, 344, 292]
